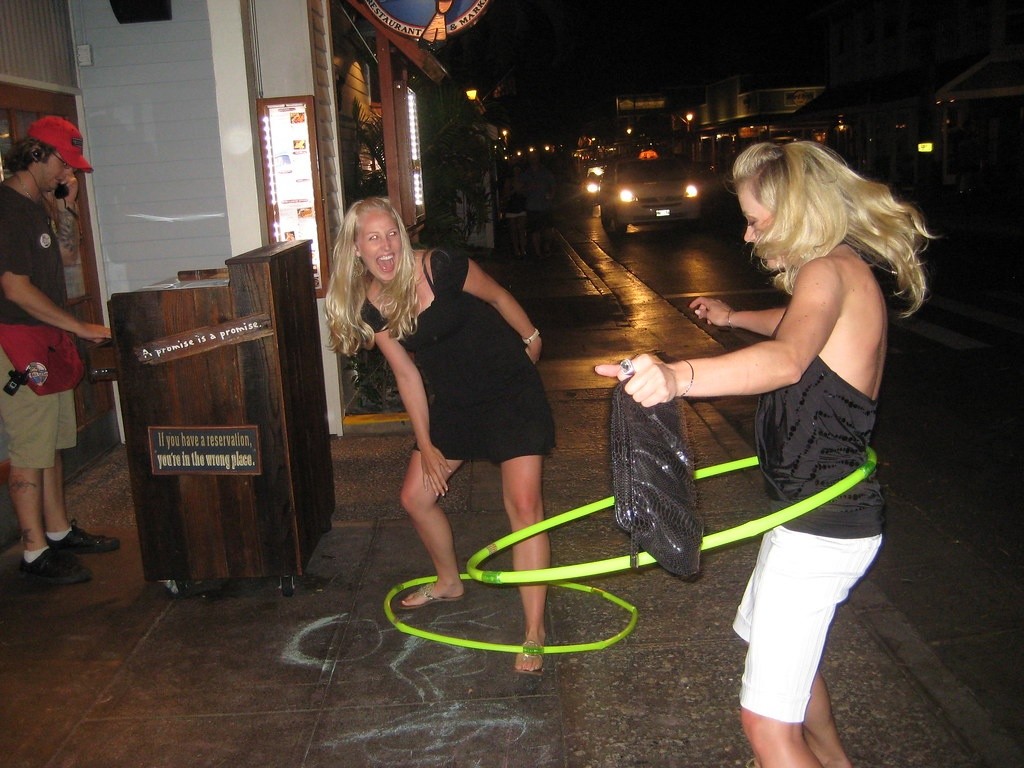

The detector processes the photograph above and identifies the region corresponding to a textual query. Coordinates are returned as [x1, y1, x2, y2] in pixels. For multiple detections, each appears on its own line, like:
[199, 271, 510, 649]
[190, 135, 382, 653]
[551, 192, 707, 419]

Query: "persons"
[322, 197, 555, 671]
[0, 115, 120, 583]
[505, 152, 558, 259]
[594, 141, 927, 768]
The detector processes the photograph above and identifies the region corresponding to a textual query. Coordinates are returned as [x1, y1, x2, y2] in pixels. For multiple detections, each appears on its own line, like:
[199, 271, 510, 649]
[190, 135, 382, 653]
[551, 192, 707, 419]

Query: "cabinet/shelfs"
[88, 240, 333, 597]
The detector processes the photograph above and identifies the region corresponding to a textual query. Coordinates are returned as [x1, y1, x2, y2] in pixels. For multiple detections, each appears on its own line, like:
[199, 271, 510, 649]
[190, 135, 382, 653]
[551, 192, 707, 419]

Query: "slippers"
[514, 640, 545, 676]
[399, 583, 464, 610]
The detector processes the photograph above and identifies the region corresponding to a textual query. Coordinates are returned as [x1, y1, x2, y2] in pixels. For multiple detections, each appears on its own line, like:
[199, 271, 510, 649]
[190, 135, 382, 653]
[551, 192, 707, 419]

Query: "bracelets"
[522, 328, 540, 344]
[728, 309, 736, 328]
[680, 360, 694, 396]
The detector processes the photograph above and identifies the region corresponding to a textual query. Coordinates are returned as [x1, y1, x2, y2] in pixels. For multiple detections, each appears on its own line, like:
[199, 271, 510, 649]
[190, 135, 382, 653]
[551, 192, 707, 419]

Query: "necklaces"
[14, 173, 32, 199]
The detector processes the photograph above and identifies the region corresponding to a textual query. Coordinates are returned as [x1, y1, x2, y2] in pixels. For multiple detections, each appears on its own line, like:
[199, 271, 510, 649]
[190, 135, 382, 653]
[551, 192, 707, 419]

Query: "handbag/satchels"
[609, 373, 705, 583]
[0, 323, 85, 395]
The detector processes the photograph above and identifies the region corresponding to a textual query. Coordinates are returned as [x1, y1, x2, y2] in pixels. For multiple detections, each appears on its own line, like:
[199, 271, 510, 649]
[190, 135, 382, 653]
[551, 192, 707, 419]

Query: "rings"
[620, 359, 636, 375]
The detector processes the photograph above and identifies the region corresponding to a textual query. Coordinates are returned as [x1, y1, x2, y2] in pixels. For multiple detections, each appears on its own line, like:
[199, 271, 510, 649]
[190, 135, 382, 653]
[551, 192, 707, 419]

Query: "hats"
[28, 115, 92, 174]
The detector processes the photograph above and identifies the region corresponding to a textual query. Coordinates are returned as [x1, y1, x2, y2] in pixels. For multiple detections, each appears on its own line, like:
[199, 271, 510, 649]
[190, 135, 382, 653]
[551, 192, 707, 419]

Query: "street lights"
[686, 112, 695, 160]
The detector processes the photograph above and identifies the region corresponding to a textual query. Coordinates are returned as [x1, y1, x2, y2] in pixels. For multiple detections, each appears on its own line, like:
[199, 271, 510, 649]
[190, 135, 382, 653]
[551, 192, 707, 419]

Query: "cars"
[581, 160, 607, 215]
[599, 150, 702, 233]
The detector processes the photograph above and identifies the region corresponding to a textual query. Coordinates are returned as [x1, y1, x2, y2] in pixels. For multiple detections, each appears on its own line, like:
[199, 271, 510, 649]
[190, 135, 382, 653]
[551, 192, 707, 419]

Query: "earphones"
[34, 148, 42, 158]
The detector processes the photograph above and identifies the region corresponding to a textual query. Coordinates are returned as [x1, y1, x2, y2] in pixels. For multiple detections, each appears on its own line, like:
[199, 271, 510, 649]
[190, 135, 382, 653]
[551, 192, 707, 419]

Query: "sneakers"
[18, 547, 91, 586]
[45, 519, 120, 555]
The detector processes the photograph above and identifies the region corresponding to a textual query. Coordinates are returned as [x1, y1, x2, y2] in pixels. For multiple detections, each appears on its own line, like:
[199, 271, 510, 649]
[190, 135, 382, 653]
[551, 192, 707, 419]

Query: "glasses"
[50, 146, 78, 173]
